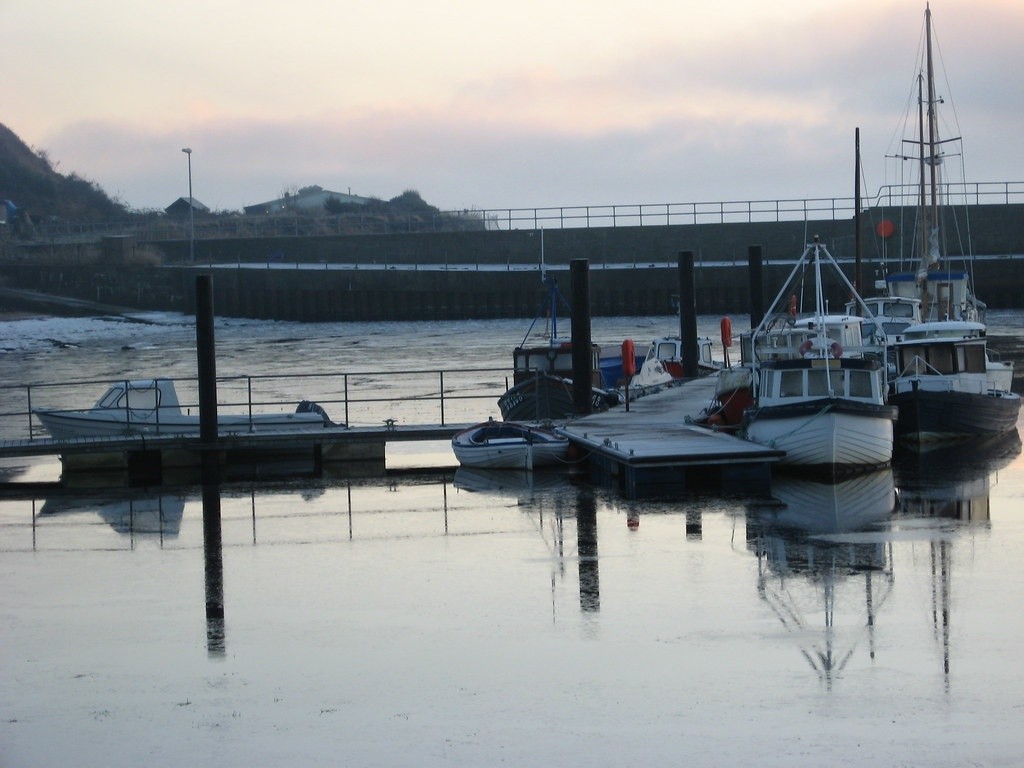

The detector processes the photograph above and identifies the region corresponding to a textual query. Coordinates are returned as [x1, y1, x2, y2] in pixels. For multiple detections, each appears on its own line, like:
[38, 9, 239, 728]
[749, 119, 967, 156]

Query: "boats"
[450, 415, 570, 472]
[31, 378, 387, 472]
[735, 235, 899, 477]
[498, 275, 725, 423]
[755, 471, 897, 688]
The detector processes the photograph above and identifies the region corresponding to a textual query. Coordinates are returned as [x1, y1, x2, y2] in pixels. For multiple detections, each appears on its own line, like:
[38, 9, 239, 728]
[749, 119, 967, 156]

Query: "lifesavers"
[799, 337, 842, 358]
[877, 219, 894, 237]
[622, 339, 636, 375]
[721, 317, 732, 347]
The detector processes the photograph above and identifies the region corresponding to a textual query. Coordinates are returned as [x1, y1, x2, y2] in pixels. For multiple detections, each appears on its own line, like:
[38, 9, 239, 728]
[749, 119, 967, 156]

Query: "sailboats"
[868, 2, 1022, 454]
